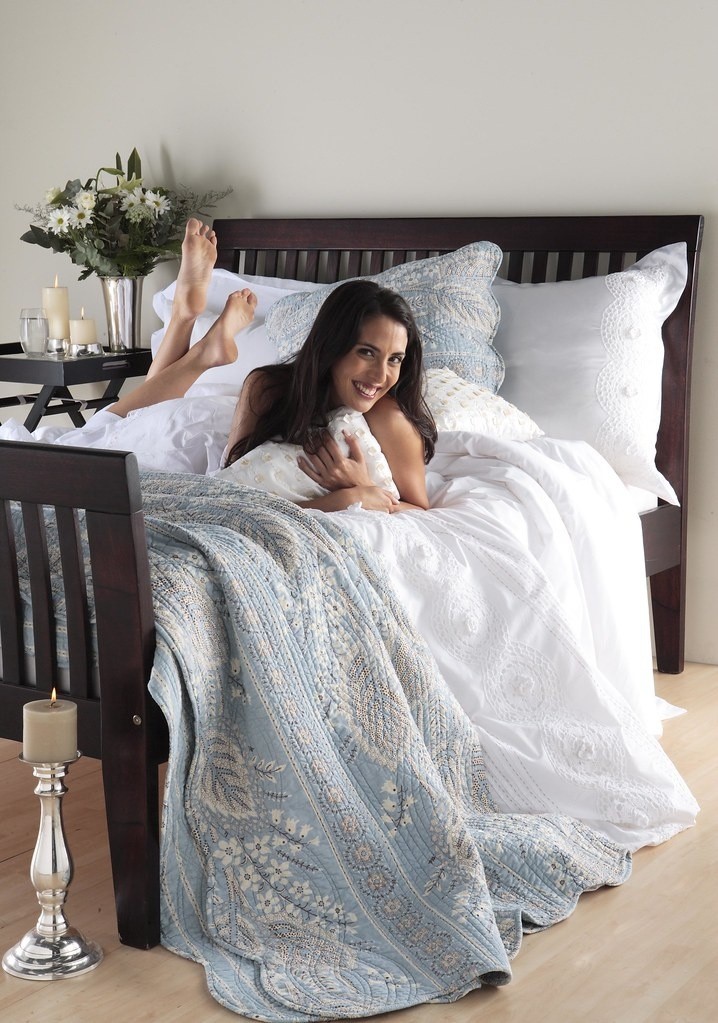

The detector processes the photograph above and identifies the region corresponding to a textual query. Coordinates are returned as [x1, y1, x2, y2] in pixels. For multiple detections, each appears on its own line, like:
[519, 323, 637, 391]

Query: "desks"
[0, 341, 156, 437]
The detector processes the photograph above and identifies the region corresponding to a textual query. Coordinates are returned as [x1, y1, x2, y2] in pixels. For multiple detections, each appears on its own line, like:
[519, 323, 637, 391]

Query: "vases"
[99, 274, 147, 354]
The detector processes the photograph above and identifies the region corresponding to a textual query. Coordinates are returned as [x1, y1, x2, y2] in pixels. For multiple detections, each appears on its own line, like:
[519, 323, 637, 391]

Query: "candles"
[23, 686, 79, 762]
[70, 306, 98, 345]
[43, 274, 72, 340]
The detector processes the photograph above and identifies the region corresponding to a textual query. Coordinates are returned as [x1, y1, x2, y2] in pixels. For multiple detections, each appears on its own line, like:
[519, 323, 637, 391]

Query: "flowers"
[16, 142, 224, 280]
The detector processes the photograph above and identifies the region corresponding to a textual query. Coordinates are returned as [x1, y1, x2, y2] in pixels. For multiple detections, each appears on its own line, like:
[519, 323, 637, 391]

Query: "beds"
[0, 213, 705, 949]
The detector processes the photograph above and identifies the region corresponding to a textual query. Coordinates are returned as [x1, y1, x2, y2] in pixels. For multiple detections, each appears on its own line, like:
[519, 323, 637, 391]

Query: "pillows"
[413, 366, 545, 445]
[264, 240, 505, 395]
[495, 242, 689, 509]
[207, 404, 401, 530]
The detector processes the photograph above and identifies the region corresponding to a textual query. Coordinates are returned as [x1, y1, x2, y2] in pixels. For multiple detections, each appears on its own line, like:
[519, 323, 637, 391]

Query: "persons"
[56, 218, 430, 514]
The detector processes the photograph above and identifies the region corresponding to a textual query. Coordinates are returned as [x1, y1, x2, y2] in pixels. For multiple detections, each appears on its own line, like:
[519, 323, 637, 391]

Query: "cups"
[18, 308, 50, 358]
